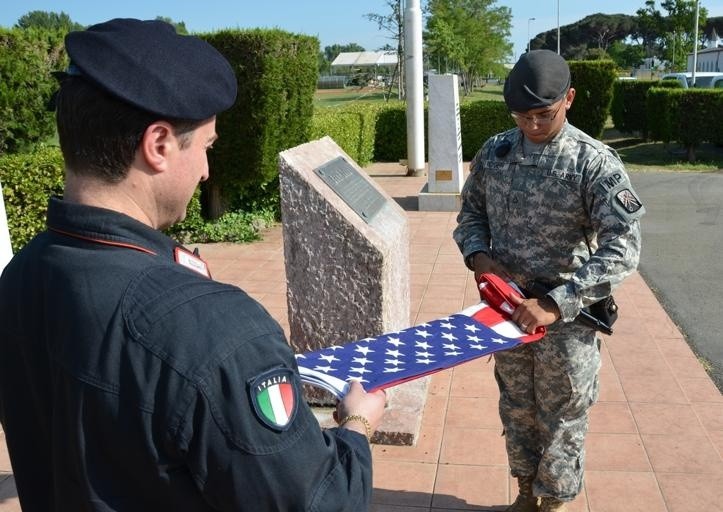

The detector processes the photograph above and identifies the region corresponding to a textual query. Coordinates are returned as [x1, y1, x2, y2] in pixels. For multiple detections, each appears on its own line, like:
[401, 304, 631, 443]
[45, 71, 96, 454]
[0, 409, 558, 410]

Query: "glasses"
[503, 100, 564, 124]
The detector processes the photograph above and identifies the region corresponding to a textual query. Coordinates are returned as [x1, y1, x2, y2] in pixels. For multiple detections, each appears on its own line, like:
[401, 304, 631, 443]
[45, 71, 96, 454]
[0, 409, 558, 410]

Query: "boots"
[504, 476, 569, 512]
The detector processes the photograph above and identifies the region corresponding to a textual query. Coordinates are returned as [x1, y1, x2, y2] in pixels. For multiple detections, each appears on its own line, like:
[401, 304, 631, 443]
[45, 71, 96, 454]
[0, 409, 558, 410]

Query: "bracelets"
[340, 414, 372, 442]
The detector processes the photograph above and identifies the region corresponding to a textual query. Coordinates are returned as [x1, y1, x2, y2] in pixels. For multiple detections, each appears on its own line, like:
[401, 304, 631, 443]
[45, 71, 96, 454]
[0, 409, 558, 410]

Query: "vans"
[661, 72, 723, 87]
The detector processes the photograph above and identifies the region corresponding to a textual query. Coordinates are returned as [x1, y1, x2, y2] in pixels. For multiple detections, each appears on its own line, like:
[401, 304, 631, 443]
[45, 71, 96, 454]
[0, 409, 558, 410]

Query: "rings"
[521, 323, 527, 328]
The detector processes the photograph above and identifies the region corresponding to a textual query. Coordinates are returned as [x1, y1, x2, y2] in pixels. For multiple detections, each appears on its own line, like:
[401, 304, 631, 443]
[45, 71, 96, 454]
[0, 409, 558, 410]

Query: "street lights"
[528, 17, 536, 54]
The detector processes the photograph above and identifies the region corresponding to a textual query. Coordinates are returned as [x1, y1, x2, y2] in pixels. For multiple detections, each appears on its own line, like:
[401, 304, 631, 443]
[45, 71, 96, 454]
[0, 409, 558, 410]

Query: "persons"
[451, 48, 646, 512]
[0, 17, 389, 511]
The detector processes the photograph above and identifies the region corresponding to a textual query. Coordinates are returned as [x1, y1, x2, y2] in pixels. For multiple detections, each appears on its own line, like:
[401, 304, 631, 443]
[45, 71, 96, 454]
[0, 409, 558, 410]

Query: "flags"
[295, 271, 546, 402]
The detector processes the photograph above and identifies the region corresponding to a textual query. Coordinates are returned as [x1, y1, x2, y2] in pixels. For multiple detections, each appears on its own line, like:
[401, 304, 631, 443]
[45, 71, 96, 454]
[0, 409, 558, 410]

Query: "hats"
[64, 18, 239, 121]
[503, 49, 572, 112]
